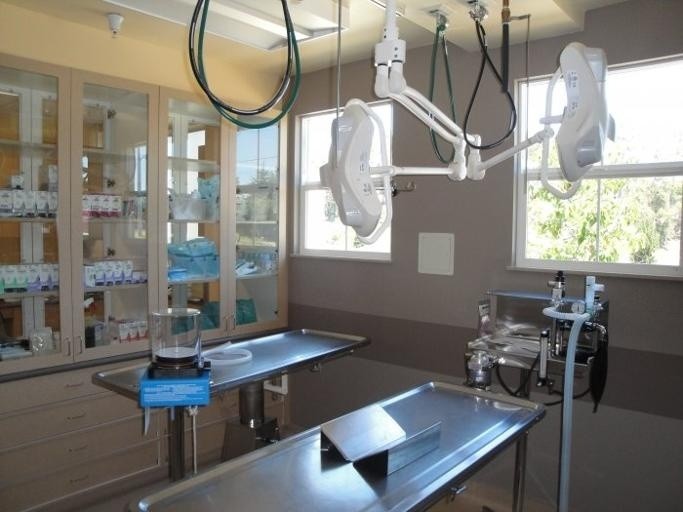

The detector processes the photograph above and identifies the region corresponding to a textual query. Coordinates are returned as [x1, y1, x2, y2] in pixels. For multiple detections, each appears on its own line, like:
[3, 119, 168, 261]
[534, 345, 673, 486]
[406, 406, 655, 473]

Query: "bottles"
[467, 349, 488, 390]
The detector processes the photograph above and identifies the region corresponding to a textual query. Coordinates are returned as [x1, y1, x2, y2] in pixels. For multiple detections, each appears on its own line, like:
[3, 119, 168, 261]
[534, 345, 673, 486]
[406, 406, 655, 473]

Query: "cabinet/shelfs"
[158, 85, 289, 347]
[0, 54, 158, 374]
[183, 374, 291, 470]
[0, 357, 168, 511]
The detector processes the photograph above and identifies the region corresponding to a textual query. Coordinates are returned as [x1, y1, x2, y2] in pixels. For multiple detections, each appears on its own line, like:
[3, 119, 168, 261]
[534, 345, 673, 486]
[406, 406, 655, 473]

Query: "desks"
[91, 328, 372, 481]
[125, 378, 549, 512]
